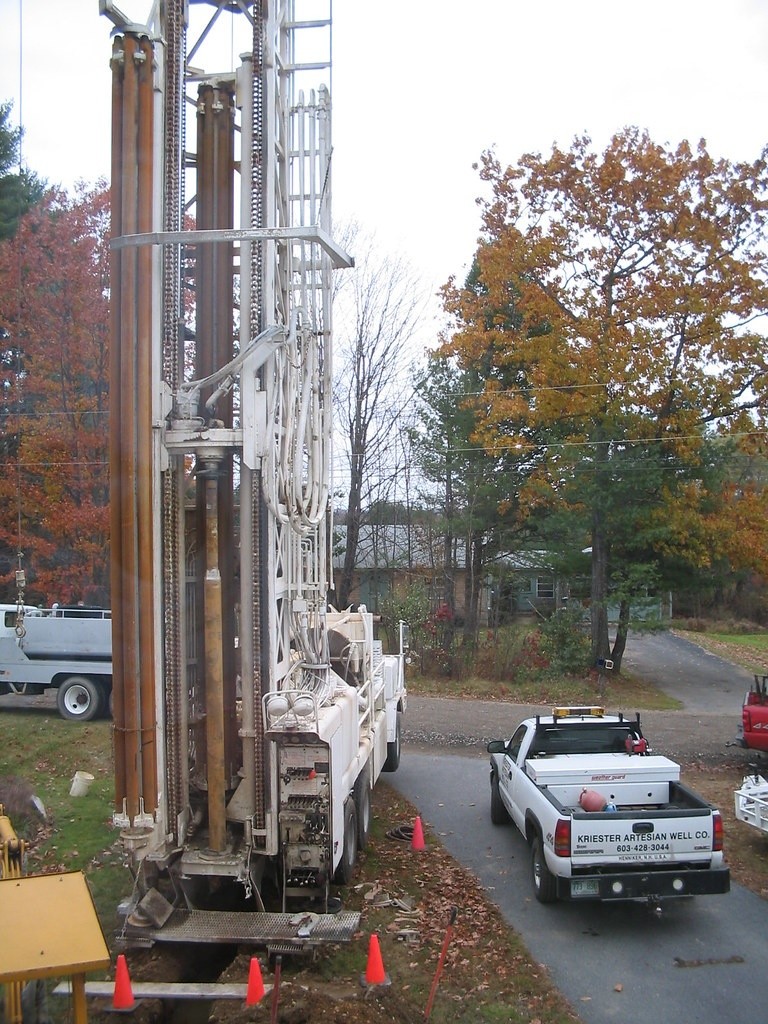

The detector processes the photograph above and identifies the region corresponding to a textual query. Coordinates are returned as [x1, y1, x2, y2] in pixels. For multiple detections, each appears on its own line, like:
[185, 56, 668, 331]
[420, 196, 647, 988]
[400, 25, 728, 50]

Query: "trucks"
[0, 602, 115, 724]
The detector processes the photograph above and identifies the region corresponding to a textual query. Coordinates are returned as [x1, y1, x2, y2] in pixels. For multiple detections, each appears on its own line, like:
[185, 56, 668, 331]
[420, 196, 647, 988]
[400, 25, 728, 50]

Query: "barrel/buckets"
[69, 771, 94, 797]
[605, 802, 617, 812]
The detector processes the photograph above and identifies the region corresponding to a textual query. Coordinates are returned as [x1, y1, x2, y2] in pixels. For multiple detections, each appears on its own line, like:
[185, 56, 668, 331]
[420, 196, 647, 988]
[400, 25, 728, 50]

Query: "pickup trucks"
[725, 674, 768, 752]
[484, 706, 730, 918]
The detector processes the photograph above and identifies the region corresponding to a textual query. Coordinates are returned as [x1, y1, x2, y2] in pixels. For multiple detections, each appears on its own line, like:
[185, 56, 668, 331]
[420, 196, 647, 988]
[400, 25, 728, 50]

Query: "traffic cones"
[240, 957, 267, 1012]
[103, 954, 143, 1015]
[358, 934, 392, 989]
[409, 816, 428, 854]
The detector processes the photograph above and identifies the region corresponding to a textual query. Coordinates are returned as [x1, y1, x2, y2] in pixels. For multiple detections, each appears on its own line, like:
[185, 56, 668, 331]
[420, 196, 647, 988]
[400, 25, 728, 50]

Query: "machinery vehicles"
[98, 0, 407, 950]
